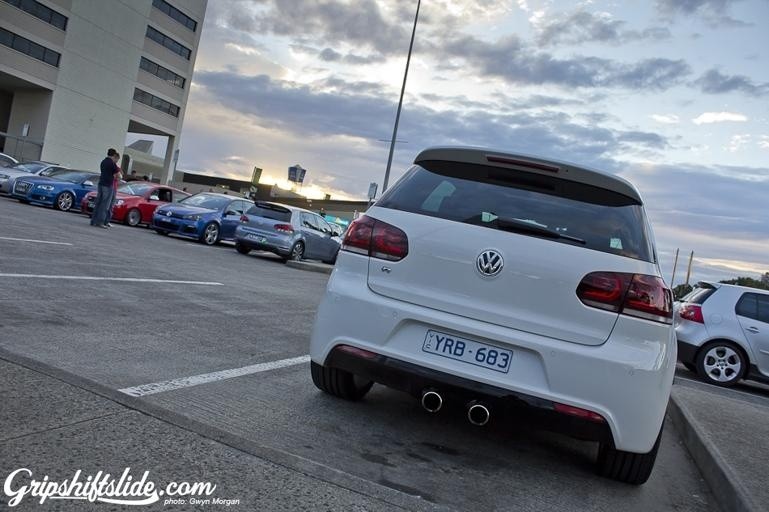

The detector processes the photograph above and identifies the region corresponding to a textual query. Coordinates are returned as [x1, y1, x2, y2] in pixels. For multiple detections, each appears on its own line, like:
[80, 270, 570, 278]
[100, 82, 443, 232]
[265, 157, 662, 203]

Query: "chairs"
[438, 189, 512, 218]
[566, 207, 629, 249]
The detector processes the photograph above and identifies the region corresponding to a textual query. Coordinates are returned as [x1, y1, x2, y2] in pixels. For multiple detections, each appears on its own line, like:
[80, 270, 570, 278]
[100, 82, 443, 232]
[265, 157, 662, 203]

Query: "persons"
[90, 147, 151, 229]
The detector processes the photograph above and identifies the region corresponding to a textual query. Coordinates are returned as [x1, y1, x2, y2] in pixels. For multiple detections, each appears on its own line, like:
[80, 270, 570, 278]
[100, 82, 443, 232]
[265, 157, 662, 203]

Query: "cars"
[672, 280, 769, 388]
[308, 145, 680, 483]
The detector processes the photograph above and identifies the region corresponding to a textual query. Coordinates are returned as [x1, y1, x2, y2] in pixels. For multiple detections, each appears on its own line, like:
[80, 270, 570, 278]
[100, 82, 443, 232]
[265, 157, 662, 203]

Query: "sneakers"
[91, 220, 112, 229]
